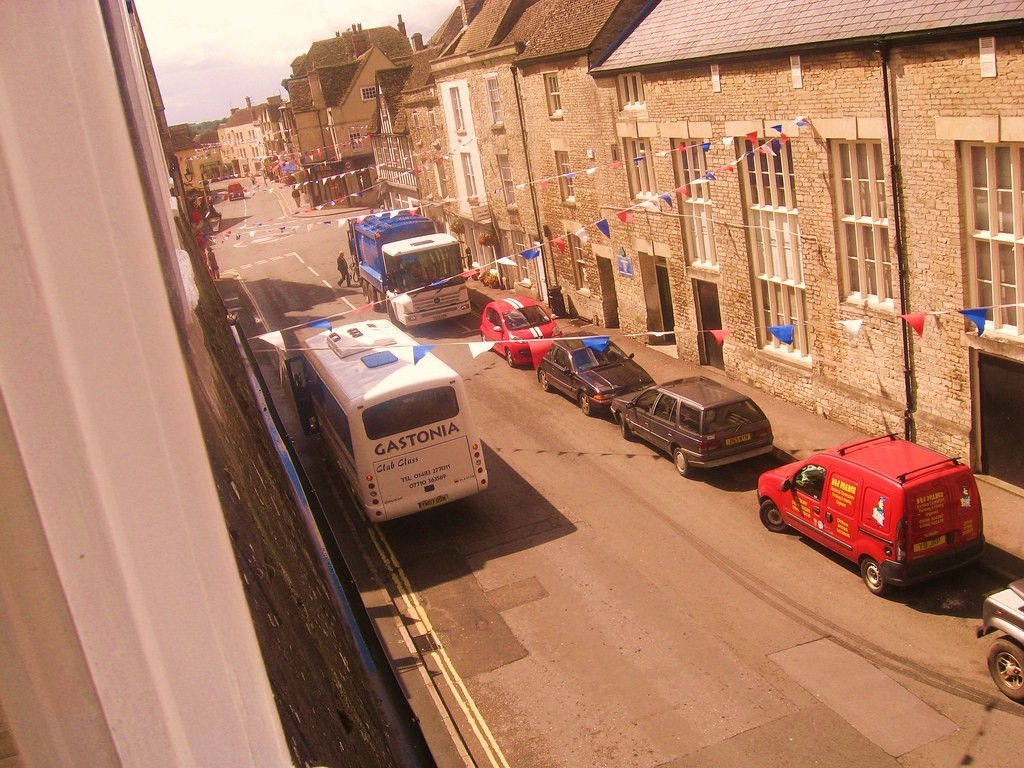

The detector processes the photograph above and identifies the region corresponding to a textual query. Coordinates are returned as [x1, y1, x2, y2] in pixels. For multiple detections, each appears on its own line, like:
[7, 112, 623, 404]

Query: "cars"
[480, 296, 566, 369]
[757, 431, 988, 597]
[536, 330, 657, 416]
[610, 375, 774, 479]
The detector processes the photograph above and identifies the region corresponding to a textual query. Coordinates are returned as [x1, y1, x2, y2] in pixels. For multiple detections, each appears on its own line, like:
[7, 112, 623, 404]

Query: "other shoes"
[347, 285, 351, 287]
[337, 282, 342, 287]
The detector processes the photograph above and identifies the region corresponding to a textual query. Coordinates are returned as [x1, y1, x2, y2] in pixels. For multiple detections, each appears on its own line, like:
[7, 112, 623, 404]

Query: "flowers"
[479, 270, 498, 289]
[479, 230, 496, 248]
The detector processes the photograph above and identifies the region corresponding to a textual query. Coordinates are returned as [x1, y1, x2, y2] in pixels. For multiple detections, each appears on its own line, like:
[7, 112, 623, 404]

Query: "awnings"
[272, 160, 285, 172]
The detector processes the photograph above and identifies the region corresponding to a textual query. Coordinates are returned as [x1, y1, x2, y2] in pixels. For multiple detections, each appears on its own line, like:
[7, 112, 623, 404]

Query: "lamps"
[180, 168, 195, 186]
[184, 202, 222, 242]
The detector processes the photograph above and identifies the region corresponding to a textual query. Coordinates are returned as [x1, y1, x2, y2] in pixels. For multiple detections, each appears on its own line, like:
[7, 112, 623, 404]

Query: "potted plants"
[449, 217, 465, 235]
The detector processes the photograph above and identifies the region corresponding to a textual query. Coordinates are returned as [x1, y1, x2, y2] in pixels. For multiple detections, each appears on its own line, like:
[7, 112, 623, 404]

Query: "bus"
[284, 318, 489, 524]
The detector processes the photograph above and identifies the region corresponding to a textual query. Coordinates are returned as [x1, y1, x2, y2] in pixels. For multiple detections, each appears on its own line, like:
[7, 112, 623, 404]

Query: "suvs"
[227, 182, 245, 201]
[975, 578, 1024, 706]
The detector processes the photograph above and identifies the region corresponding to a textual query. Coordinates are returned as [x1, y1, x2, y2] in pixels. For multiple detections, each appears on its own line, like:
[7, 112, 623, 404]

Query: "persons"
[337, 252, 351, 287]
[251, 173, 255, 184]
[291, 185, 301, 208]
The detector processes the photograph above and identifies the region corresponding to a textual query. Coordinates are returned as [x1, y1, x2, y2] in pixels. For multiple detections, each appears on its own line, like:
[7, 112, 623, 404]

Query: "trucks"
[347, 209, 472, 328]
